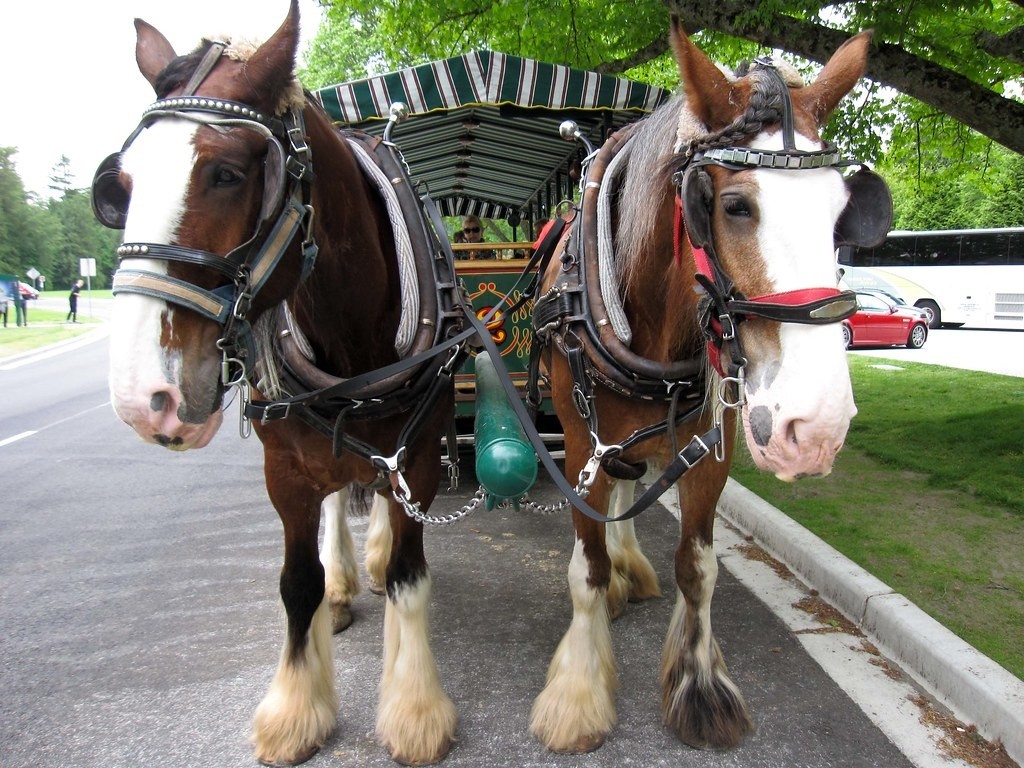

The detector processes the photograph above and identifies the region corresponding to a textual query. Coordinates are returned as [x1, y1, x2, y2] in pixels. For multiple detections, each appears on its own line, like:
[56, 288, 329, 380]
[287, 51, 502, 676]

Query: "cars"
[12, 282, 40, 300]
[839, 287, 930, 351]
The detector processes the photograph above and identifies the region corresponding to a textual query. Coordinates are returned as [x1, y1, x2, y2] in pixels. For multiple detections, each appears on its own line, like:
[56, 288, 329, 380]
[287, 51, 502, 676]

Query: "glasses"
[463, 227, 481, 234]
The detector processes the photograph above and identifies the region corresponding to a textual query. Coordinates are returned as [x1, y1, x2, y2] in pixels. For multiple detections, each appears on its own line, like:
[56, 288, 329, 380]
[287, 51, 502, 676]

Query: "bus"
[834, 227, 1024, 332]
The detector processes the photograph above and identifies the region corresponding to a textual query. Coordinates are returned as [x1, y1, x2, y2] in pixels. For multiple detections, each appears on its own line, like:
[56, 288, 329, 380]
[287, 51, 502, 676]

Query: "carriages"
[94, 0, 897, 767]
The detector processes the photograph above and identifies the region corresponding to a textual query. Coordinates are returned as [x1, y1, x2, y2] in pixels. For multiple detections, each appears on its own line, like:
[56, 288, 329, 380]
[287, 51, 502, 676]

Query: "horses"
[106, 0, 457, 767]
[528, 14, 872, 756]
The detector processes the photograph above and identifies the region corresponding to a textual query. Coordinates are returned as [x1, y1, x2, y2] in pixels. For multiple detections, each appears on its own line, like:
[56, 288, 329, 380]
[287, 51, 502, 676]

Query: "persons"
[524, 218, 548, 260]
[0, 280, 83, 327]
[455, 216, 496, 259]
